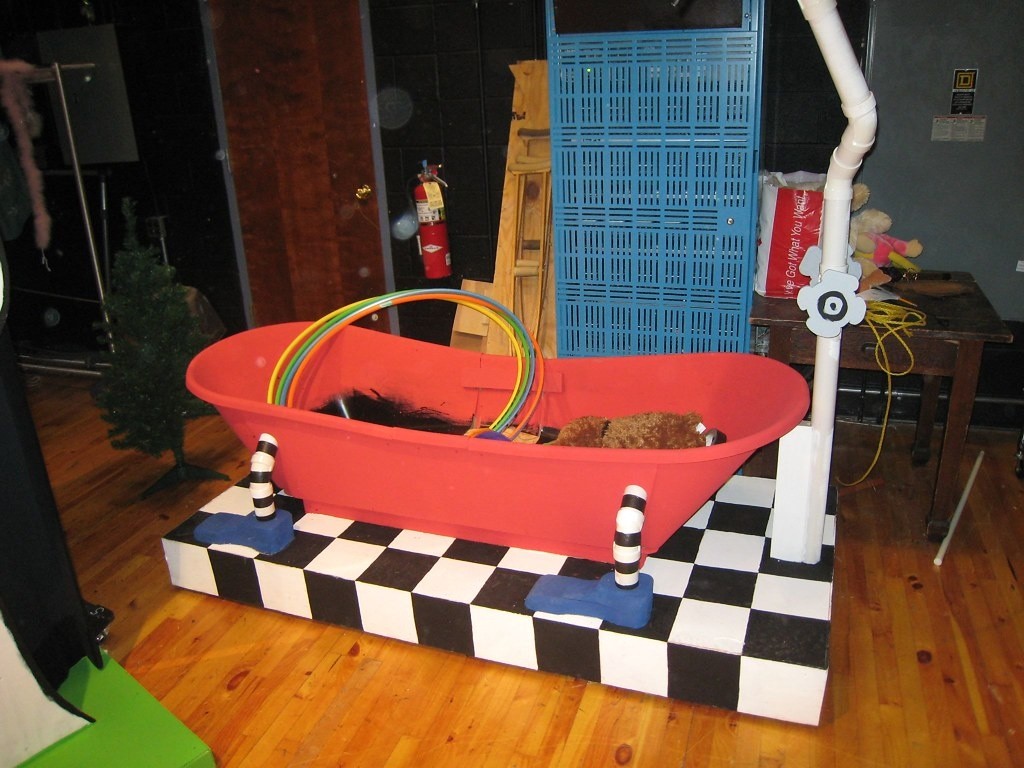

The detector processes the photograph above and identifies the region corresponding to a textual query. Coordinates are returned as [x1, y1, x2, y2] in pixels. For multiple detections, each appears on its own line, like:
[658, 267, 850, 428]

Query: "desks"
[750, 270, 1014, 542]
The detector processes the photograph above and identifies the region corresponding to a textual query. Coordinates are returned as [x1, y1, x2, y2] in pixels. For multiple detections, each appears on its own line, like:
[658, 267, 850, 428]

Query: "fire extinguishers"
[413, 159, 456, 281]
